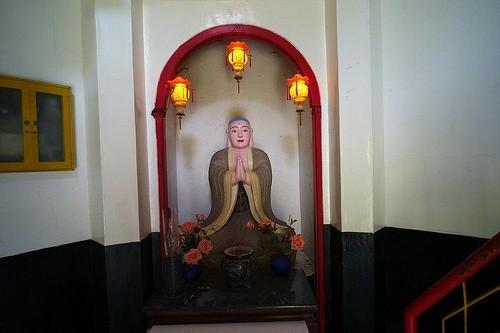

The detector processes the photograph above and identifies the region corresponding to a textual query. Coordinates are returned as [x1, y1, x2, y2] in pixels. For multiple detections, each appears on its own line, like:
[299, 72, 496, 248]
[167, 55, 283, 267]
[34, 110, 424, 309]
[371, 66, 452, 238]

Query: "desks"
[140, 268, 319, 333]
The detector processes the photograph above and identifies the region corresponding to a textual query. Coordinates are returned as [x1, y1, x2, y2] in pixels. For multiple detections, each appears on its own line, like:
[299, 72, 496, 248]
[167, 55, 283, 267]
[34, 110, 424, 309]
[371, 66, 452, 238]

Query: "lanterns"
[167, 76, 194, 129]
[226, 41, 252, 94]
[287, 74, 309, 125]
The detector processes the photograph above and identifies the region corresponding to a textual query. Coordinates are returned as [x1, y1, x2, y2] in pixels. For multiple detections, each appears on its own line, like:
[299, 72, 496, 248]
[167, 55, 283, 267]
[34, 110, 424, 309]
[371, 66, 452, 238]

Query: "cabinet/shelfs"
[0, 75, 75, 173]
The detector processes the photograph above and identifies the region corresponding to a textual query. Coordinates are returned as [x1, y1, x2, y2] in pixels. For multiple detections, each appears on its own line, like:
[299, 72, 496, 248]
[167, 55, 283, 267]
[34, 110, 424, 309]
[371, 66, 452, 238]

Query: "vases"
[183, 263, 203, 279]
[270, 254, 293, 274]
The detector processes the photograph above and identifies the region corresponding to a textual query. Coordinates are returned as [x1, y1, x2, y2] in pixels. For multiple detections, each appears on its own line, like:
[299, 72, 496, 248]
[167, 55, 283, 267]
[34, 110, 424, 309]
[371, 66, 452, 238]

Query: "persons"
[199, 118, 290, 264]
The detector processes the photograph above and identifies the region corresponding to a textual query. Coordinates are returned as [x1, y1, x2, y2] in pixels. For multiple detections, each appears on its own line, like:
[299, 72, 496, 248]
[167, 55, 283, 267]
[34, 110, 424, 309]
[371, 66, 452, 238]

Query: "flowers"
[241, 216, 303, 258]
[180, 214, 214, 265]
